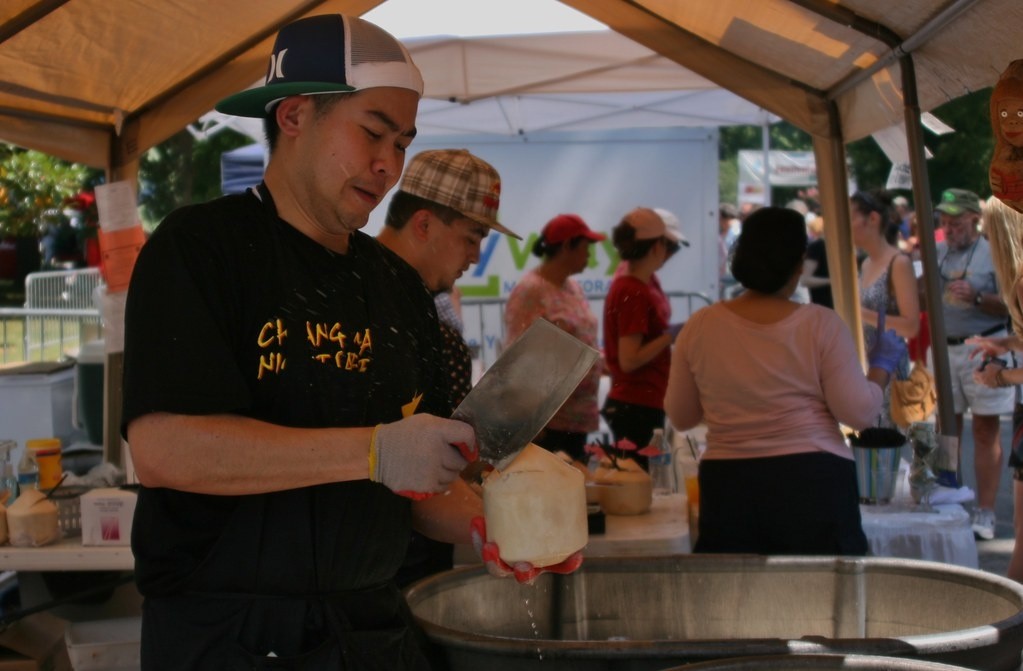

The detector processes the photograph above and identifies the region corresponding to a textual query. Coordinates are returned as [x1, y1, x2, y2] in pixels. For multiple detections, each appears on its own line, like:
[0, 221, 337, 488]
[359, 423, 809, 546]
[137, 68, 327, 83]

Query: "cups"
[847, 427, 908, 506]
[873, 468, 906, 513]
[681, 457, 700, 529]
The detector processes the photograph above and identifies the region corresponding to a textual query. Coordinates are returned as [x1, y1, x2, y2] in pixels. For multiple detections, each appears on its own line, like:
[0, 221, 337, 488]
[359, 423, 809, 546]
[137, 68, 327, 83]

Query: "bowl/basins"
[593, 481, 654, 515]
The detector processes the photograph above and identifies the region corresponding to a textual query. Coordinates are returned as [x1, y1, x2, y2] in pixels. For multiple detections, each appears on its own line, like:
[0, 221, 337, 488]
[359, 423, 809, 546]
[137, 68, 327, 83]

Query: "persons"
[120, 12, 583, 671]
[990, 59, 1023, 214]
[503, 206, 690, 473]
[663, 207, 909, 556]
[718, 186, 1023, 585]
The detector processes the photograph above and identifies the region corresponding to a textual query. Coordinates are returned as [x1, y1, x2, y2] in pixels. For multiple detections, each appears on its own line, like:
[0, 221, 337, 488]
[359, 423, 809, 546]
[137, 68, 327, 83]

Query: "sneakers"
[971, 505, 996, 539]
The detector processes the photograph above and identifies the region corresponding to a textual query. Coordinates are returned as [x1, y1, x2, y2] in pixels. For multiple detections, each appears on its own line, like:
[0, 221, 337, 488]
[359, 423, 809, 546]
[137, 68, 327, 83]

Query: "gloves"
[868, 327, 908, 371]
[664, 323, 683, 342]
[369, 412, 477, 499]
[470, 514, 583, 583]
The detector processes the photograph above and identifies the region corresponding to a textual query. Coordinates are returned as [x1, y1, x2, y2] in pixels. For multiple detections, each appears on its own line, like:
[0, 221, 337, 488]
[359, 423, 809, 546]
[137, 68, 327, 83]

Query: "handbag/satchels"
[892, 358, 935, 428]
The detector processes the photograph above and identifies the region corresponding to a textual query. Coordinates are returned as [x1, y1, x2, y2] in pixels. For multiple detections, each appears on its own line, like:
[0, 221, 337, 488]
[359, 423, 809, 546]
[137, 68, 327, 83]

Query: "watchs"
[996, 368, 1009, 387]
[976, 292, 983, 306]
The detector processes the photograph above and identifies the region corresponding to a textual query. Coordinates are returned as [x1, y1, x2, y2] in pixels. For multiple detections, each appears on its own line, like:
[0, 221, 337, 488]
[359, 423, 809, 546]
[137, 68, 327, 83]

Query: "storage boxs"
[64, 618, 142, 671]
[81, 488, 138, 546]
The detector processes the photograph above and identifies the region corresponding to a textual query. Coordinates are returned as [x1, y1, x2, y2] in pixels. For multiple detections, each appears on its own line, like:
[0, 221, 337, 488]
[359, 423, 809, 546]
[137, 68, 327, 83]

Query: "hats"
[621, 207, 679, 243]
[217, 13, 424, 116]
[401, 148, 524, 239]
[542, 217, 606, 246]
[934, 188, 982, 217]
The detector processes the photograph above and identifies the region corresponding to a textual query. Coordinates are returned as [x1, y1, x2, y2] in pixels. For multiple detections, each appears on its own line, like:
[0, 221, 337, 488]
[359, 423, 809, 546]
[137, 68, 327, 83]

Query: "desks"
[867, 499, 979, 570]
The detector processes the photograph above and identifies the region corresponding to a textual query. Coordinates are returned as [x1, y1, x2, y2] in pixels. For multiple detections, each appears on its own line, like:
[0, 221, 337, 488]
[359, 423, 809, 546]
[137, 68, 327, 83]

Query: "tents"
[0, 0, 1023, 437]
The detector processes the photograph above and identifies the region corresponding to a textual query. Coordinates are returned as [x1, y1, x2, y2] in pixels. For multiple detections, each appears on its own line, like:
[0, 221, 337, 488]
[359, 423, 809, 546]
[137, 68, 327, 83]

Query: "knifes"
[448, 317, 600, 475]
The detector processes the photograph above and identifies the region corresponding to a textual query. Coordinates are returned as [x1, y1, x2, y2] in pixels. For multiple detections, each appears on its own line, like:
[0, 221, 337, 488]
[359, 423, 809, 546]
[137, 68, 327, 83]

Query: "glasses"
[939, 250, 970, 282]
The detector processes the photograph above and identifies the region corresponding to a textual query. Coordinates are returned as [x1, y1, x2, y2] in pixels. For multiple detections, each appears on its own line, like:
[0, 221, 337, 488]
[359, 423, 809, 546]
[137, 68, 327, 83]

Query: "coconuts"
[0, 487, 59, 549]
[592, 454, 653, 517]
[481, 441, 588, 569]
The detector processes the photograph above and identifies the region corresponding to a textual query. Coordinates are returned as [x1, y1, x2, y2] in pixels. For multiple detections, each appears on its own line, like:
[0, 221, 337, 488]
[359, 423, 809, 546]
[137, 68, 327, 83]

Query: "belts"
[948, 324, 1007, 345]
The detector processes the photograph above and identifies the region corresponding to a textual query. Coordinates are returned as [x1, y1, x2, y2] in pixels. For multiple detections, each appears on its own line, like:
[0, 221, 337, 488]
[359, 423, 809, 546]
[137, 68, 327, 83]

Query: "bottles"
[0, 462, 18, 507]
[646, 429, 673, 498]
[17, 446, 39, 495]
